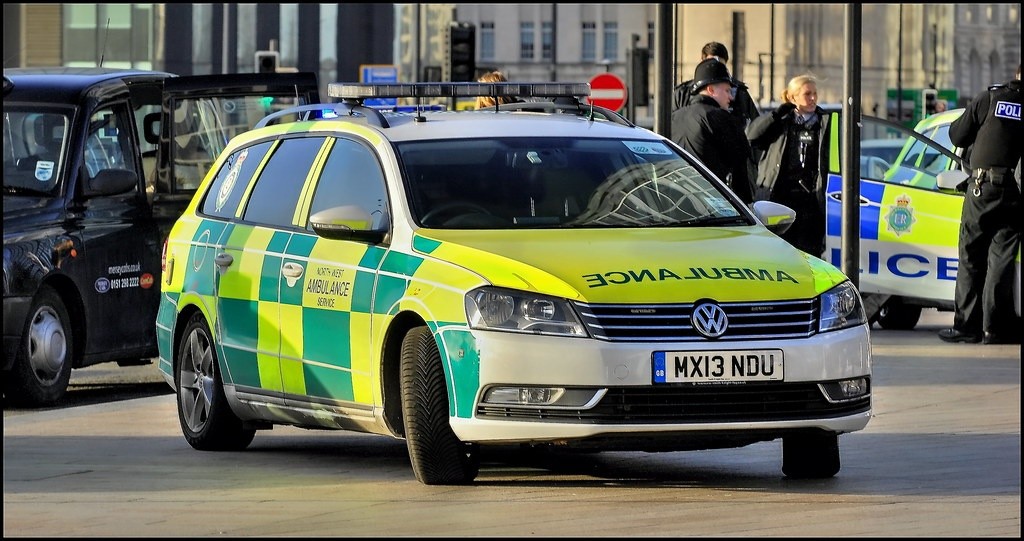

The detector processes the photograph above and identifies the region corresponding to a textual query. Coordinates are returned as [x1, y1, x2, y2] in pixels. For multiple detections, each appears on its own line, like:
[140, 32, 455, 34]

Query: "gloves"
[773, 102, 796, 118]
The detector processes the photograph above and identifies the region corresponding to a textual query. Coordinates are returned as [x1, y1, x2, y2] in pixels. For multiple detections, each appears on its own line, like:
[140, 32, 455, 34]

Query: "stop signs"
[586, 73, 627, 113]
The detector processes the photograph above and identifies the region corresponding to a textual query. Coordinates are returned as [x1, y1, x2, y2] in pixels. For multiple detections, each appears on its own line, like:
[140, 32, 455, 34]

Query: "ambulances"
[156, 82, 873, 486]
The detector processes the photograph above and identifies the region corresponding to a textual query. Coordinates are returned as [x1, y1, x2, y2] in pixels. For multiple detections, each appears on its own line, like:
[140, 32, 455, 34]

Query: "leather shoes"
[985, 331, 1001, 344]
[939, 327, 983, 344]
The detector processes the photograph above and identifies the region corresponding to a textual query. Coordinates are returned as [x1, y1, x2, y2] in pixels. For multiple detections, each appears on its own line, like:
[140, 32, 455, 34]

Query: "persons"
[927, 65, 1022, 344]
[309, 194, 389, 230]
[669, 41, 830, 261]
[471, 72, 524, 111]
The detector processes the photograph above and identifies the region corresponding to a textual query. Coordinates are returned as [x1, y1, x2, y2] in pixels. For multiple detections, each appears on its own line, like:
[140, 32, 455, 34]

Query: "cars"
[826, 108, 1021, 330]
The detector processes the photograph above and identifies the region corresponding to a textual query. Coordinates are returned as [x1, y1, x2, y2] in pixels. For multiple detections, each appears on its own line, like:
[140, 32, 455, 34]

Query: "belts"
[972, 170, 990, 181]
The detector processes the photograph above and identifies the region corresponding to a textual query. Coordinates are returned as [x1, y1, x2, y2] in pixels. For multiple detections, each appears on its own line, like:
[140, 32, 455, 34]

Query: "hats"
[691, 58, 739, 95]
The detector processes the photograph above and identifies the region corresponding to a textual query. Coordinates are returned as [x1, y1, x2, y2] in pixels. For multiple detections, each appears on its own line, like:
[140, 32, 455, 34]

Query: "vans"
[3, 69, 320, 410]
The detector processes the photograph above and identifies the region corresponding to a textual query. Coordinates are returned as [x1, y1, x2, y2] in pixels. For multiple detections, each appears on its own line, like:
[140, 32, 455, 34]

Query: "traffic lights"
[254, 51, 279, 73]
[922, 89, 938, 120]
[442, 26, 475, 82]
